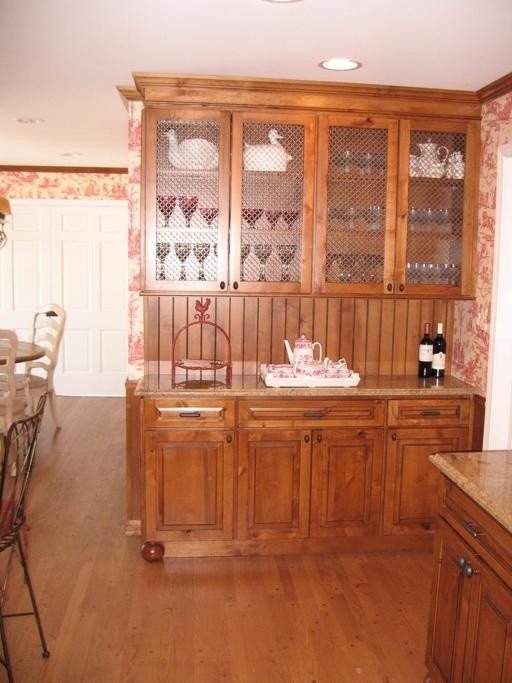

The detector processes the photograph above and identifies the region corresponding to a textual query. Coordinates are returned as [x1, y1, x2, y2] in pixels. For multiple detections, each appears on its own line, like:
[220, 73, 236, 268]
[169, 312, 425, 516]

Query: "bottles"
[417, 320, 447, 378]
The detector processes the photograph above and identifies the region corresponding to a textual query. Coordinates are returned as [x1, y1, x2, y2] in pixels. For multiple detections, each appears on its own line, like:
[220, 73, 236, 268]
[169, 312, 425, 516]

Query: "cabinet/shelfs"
[317, 113, 480, 298]
[417, 473, 511, 683]
[141, 103, 317, 296]
[383, 394, 473, 550]
[235, 396, 386, 558]
[139, 396, 235, 558]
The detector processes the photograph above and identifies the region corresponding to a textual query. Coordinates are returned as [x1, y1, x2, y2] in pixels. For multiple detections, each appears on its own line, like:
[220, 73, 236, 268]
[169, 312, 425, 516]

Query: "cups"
[407, 263, 461, 285]
[326, 368, 351, 378]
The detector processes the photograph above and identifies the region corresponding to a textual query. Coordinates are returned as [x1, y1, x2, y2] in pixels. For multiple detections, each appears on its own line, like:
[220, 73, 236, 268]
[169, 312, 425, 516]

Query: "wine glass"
[156, 194, 297, 284]
[405, 208, 464, 233]
[327, 206, 385, 233]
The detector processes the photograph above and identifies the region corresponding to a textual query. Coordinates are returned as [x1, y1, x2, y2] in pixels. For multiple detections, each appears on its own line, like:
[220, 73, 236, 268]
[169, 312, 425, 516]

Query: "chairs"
[1, 304, 68, 683]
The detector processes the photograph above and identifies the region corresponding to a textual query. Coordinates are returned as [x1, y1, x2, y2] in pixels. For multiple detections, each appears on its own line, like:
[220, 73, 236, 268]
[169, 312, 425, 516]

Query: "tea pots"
[283, 332, 326, 376]
[417, 140, 448, 177]
